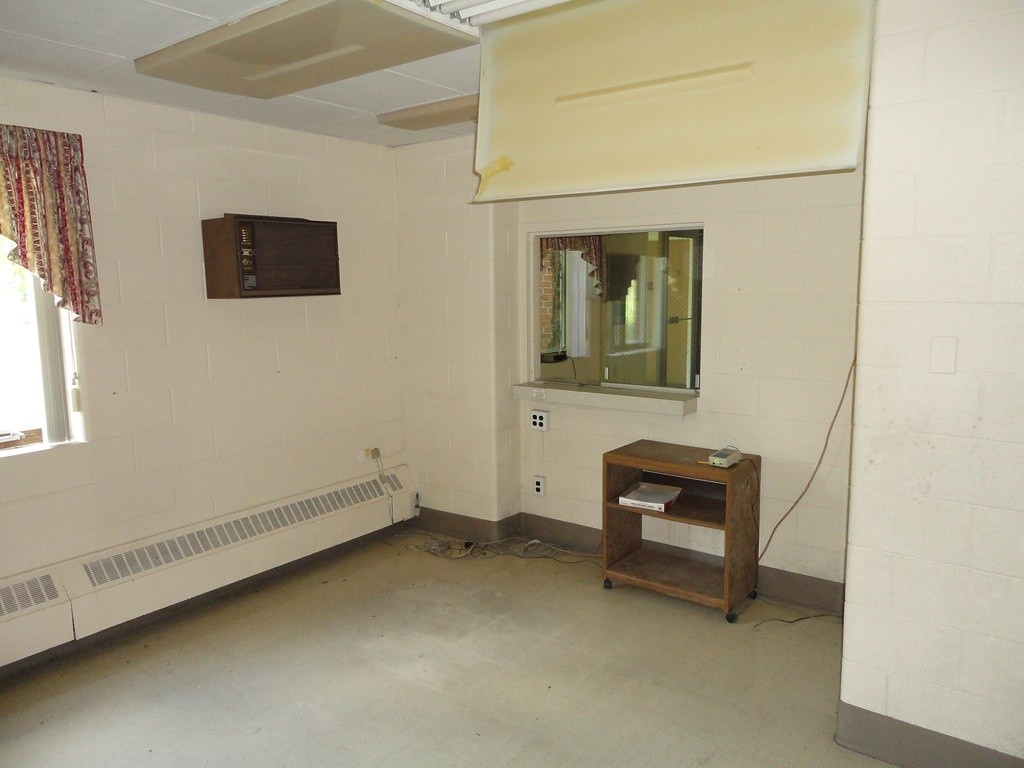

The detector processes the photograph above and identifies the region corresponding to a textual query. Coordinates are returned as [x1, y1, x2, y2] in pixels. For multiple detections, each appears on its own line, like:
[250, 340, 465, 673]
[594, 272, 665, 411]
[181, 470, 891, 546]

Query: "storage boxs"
[619, 481, 683, 513]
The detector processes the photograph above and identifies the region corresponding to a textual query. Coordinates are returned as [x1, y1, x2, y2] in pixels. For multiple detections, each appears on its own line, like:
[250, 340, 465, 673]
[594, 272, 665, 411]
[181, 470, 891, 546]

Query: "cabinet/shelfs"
[602, 439, 762, 622]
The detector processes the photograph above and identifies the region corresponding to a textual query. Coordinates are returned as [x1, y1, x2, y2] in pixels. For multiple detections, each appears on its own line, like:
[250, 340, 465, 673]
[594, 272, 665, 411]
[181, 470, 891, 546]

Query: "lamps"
[411, 0, 575, 26]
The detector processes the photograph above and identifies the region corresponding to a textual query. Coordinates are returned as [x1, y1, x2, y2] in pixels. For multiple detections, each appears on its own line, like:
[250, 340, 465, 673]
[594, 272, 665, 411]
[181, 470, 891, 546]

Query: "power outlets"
[533, 476, 546, 495]
[372, 448, 380, 460]
[531, 410, 550, 430]
